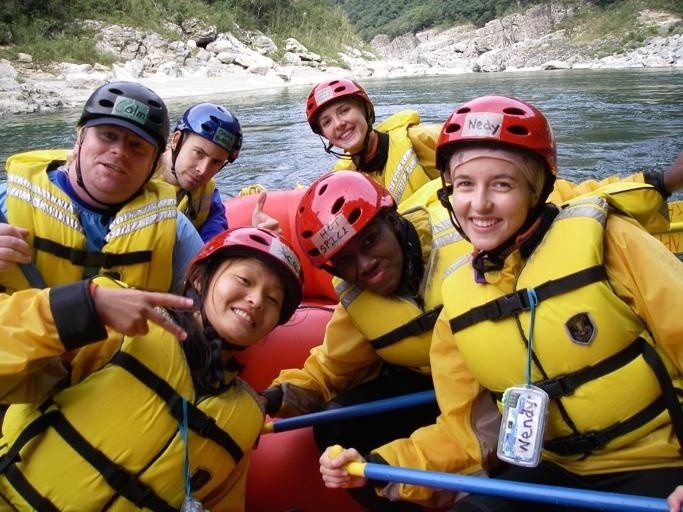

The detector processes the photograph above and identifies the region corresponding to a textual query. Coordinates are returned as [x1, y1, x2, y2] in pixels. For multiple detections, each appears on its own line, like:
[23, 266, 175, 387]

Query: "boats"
[211, 189, 369, 512]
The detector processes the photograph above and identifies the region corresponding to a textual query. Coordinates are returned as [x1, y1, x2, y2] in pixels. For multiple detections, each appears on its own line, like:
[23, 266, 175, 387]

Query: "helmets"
[188, 170, 397, 325]
[435, 96, 556, 176]
[306, 80, 375, 134]
[78, 81, 243, 163]
